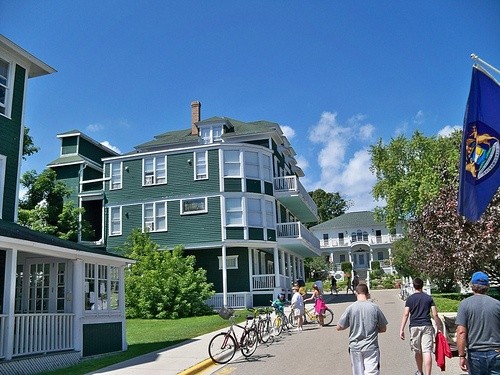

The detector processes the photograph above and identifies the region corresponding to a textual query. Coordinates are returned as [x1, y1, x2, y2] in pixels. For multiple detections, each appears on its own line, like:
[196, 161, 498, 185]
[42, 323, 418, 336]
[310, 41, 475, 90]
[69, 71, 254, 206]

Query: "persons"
[337, 284, 388, 375]
[316, 278, 323, 293]
[400, 277, 444, 375]
[271, 293, 285, 331]
[456, 272, 500, 375]
[330, 276, 338, 296]
[304, 284, 327, 327]
[291, 282, 306, 330]
[347, 276, 359, 293]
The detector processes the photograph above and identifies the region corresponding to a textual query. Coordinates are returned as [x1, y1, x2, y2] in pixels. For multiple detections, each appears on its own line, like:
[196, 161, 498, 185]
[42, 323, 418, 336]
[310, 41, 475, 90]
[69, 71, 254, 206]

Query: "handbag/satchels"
[332, 285, 336, 290]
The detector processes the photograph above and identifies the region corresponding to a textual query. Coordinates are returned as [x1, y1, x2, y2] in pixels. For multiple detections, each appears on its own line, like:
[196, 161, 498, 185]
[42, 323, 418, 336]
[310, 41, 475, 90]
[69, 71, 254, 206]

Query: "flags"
[458, 64, 500, 221]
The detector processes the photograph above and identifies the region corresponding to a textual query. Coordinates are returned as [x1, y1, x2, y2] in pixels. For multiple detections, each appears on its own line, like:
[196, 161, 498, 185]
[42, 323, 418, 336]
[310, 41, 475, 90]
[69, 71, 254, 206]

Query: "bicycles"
[247, 295, 334, 344]
[207, 305, 259, 365]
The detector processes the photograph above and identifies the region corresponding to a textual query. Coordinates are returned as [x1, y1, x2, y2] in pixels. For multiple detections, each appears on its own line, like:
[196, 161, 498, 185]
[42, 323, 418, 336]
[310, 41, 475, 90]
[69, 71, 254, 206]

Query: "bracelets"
[460, 355, 465, 357]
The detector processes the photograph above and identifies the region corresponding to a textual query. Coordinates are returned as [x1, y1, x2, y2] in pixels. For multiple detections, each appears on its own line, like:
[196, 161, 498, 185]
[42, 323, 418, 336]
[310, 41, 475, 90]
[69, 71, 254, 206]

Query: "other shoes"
[294, 328, 300, 330]
[300, 328, 303, 330]
[415, 371, 423, 375]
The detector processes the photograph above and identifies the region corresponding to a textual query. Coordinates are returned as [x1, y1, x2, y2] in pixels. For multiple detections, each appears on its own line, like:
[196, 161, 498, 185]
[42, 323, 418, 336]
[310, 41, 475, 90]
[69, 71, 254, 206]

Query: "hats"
[292, 287, 298, 289]
[471, 272, 489, 285]
